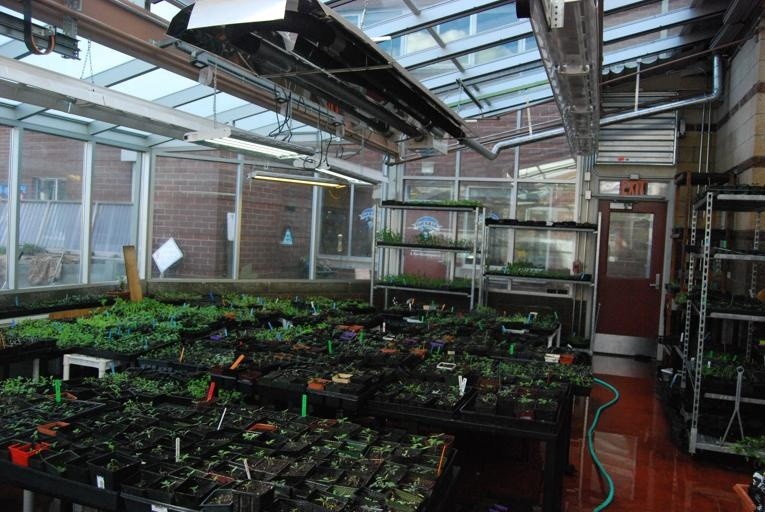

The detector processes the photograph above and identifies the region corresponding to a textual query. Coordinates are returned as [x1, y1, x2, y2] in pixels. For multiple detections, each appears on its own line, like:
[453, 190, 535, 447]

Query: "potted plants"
[0, 290, 591, 508]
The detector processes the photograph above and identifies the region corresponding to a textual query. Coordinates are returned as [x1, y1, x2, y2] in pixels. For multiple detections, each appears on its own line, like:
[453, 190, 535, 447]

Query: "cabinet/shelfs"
[372, 200, 603, 342]
[660, 166, 765, 458]
